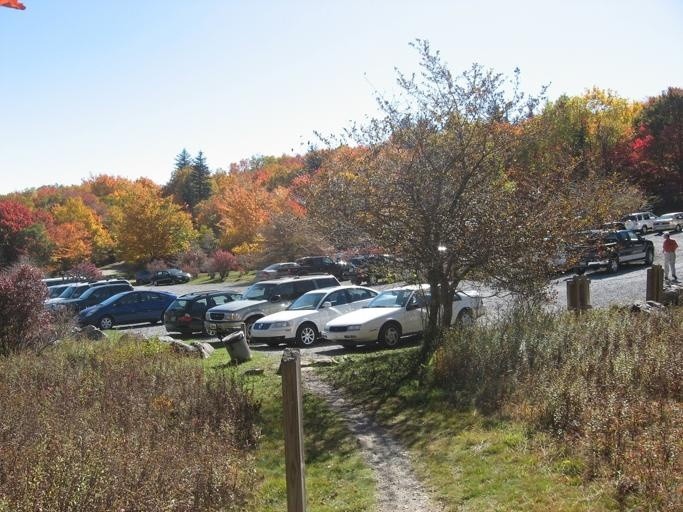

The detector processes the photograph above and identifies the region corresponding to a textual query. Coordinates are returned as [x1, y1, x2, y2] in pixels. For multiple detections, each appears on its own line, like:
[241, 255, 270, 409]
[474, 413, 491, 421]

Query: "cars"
[514, 209, 683, 274]
[133, 269, 154, 285]
[256, 254, 404, 285]
[151, 269, 190, 286]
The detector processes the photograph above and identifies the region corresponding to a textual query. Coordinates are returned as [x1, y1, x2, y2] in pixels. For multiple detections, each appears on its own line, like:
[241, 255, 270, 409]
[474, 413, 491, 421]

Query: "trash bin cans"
[564, 275, 593, 309]
[222, 330, 253, 364]
[646, 265, 665, 301]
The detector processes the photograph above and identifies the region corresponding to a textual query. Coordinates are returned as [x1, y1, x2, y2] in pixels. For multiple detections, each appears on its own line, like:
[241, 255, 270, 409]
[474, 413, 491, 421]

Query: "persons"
[660, 230, 679, 283]
